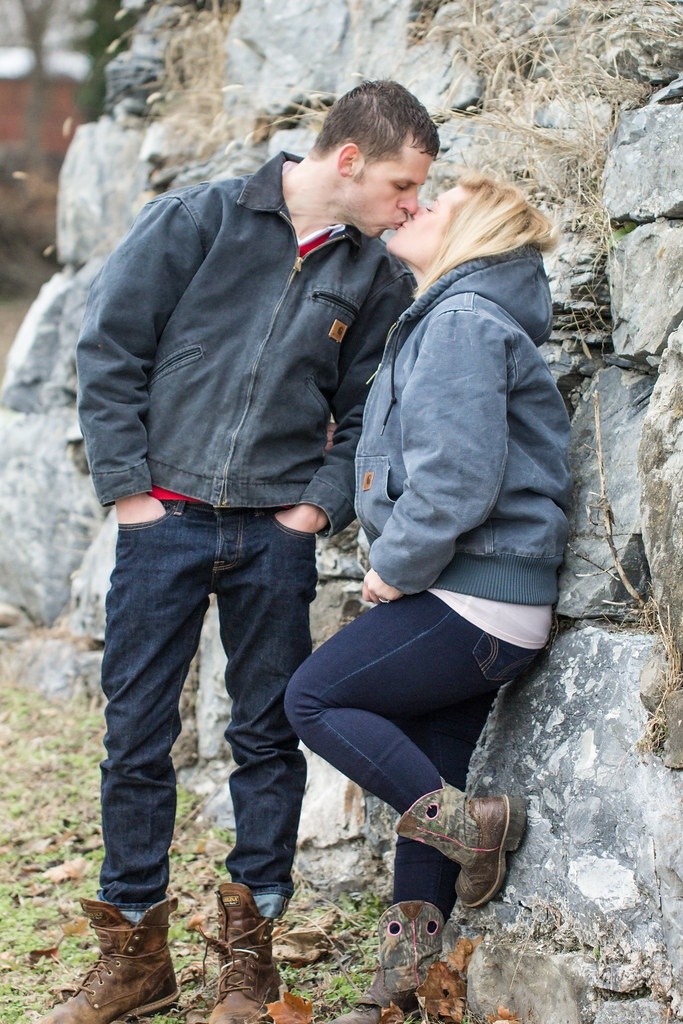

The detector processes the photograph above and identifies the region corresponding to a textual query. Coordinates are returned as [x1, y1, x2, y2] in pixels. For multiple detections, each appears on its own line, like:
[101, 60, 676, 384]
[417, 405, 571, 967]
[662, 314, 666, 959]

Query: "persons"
[34, 79, 440, 1024]
[285, 177, 573, 1024]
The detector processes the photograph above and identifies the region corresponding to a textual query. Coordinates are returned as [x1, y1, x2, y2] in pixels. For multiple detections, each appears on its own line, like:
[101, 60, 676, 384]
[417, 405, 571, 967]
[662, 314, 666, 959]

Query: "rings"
[379, 598, 390, 606]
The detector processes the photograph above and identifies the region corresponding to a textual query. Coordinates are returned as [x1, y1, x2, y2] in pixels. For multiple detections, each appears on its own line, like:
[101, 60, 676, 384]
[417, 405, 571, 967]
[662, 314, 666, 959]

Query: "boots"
[330, 900, 445, 1024]
[194, 882, 288, 1024]
[394, 776, 527, 909]
[32, 892, 181, 1024]
